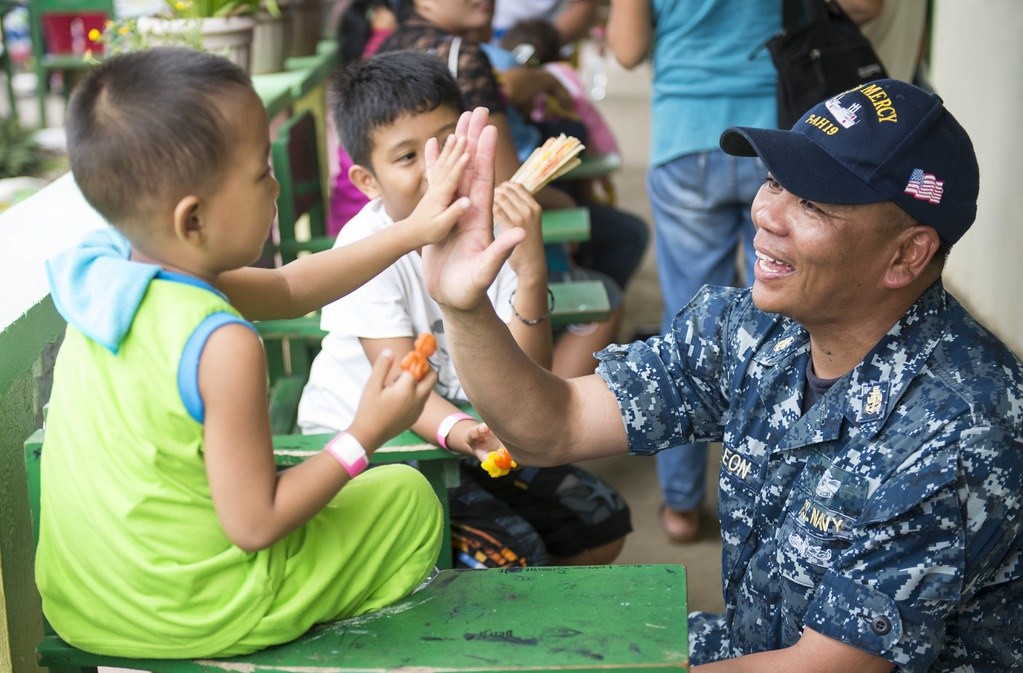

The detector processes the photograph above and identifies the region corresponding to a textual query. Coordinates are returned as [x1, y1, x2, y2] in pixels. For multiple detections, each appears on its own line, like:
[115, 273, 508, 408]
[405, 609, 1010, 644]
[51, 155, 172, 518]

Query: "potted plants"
[249, 1, 287, 74]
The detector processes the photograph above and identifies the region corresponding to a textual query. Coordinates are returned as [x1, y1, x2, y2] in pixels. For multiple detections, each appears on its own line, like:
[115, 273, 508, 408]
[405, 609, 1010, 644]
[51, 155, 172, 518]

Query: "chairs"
[27, 0, 113, 130]
[0, 0, 692, 673]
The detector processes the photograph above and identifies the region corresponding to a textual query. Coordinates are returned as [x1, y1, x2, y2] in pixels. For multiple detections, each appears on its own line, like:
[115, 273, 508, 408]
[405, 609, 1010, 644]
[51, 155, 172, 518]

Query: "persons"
[422, 79, 1023, 672]
[327, 0, 650, 379]
[605, 0, 884, 548]
[31, 43, 472, 660]
[298, 49, 631, 570]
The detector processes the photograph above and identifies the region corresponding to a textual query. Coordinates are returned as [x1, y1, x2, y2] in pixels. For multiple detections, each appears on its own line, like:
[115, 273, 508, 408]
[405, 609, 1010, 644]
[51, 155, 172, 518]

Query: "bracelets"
[436, 412, 479, 456]
[508, 287, 555, 326]
[324, 431, 369, 479]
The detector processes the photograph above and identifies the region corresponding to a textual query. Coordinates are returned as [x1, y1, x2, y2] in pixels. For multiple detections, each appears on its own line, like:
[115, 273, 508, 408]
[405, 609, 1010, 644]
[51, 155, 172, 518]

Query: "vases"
[138, 16, 255, 72]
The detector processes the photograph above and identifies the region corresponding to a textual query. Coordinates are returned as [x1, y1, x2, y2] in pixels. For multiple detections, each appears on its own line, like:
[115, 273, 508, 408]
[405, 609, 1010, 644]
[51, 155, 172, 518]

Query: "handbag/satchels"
[765, 1, 889, 131]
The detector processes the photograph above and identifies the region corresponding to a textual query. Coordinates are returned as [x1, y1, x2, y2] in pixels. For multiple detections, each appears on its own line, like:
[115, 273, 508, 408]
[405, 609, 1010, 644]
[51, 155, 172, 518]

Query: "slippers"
[659, 499, 703, 543]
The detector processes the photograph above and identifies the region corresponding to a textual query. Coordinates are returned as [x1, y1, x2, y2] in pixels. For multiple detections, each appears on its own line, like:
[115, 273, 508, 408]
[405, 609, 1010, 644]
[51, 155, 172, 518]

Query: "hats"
[719, 78, 980, 245]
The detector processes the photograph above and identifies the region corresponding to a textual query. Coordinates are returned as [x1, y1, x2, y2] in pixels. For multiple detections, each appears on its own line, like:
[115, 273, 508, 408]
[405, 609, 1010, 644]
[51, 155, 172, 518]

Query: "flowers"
[85, 0, 281, 65]
